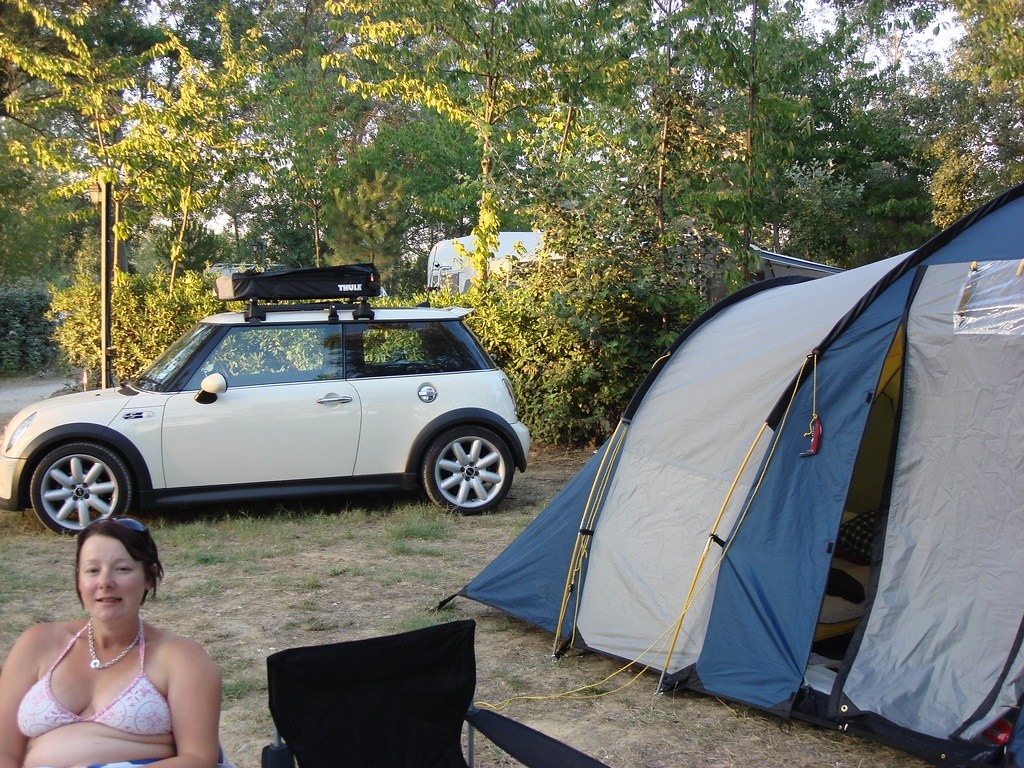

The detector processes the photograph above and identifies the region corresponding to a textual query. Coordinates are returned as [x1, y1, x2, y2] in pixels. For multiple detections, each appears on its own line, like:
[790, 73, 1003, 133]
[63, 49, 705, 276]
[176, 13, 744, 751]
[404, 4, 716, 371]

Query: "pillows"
[840, 507, 879, 562]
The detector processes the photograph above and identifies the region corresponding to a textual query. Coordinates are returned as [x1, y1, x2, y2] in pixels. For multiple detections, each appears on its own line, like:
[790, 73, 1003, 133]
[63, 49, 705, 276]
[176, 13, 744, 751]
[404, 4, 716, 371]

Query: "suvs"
[0, 260, 533, 541]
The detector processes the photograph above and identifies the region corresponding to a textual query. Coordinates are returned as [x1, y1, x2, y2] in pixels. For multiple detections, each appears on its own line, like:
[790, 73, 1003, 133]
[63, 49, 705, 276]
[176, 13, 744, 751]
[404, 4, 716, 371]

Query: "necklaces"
[88, 618, 143, 669]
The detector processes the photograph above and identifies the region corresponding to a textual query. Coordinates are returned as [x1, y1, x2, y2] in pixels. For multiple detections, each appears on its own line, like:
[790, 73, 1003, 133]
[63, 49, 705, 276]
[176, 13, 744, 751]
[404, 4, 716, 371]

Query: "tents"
[456, 182, 1024, 768]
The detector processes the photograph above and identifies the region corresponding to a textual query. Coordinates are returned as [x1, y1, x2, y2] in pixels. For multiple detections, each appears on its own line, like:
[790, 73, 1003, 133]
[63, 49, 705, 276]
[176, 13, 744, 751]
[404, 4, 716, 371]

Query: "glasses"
[89, 518, 151, 549]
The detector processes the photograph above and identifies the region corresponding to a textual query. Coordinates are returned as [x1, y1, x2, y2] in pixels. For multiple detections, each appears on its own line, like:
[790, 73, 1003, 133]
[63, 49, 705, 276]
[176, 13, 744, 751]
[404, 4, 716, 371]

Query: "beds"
[812, 510, 871, 659]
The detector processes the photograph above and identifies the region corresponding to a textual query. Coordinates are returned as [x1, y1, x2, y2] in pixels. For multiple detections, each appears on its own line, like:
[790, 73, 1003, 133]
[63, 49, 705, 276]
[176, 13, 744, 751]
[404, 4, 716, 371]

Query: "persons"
[0, 518, 234, 768]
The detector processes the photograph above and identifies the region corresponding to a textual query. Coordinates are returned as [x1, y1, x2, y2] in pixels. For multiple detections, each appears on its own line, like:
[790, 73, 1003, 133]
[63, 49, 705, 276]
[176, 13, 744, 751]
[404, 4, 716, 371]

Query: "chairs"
[262, 619, 610, 768]
